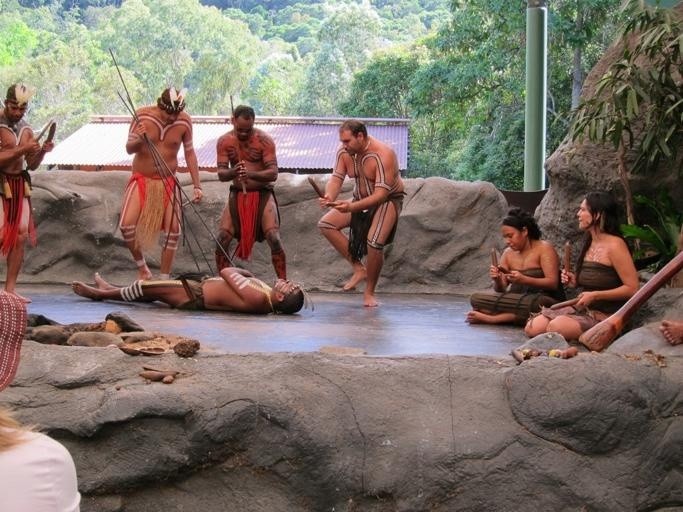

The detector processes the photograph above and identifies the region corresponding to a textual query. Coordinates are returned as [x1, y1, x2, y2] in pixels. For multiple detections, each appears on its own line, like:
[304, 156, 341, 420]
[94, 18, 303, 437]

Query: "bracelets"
[193, 185, 202, 190]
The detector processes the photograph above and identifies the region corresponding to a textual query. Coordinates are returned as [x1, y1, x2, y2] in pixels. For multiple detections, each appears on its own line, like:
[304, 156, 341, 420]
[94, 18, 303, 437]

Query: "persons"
[463, 202, 565, 326]
[0, 82, 55, 306]
[658, 319, 682, 346]
[212, 104, 290, 281]
[314, 118, 404, 307]
[69, 265, 304, 316]
[0, 290, 82, 512]
[522, 189, 640, 342]
[117, 87, 203, 280]
